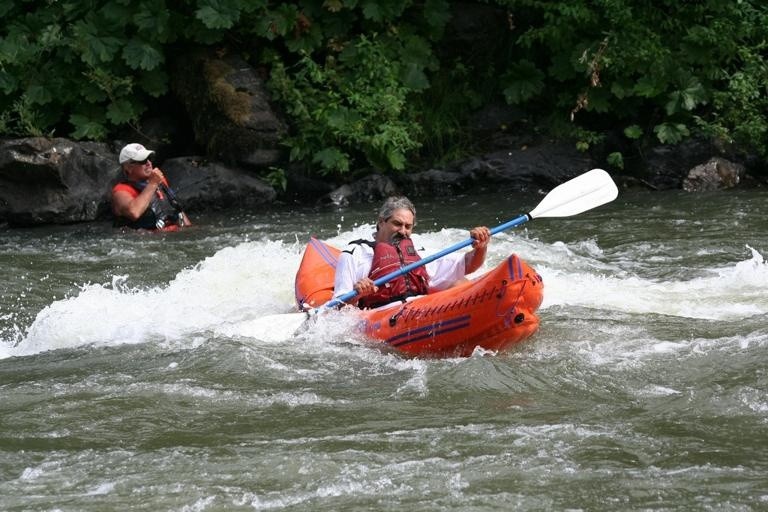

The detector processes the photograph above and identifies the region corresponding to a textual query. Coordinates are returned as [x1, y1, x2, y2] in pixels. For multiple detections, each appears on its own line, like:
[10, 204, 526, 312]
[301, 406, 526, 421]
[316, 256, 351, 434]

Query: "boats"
[293, 237, 545, 358]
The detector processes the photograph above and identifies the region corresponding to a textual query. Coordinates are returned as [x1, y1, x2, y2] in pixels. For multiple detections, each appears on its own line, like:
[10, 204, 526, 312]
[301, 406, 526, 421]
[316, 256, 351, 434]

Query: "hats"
[119, 142, 156, 165]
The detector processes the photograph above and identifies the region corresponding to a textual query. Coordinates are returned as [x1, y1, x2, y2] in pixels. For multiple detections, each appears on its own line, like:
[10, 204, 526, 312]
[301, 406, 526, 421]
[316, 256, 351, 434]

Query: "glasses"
[131, 156, 150, 165]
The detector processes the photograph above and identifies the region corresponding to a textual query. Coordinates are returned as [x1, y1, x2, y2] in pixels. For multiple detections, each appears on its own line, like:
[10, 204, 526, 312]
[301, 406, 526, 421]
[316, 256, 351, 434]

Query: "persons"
[333, 192, 491, 310]
[110, 141, 192, 234]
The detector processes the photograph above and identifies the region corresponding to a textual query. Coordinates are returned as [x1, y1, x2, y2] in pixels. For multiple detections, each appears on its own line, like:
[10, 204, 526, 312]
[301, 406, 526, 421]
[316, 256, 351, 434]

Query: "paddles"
[237, 168, 618, 341]
